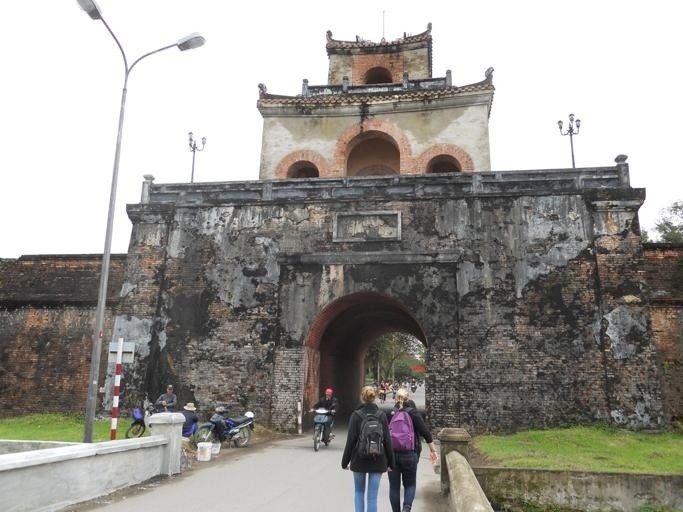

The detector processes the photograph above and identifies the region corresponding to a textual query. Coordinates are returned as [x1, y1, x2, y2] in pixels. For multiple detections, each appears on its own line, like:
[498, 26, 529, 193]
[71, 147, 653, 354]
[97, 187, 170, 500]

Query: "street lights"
[557, 114, 581, 168]
[188, 132, 206, 183]
[77, 0, 205, 443]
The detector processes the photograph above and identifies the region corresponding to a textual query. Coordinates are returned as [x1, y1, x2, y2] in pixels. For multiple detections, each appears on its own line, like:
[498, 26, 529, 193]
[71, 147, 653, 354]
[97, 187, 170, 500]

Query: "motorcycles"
[411, 385, 417, 393]
[309, 407, 336, 451]
[126, 392, 254, 448]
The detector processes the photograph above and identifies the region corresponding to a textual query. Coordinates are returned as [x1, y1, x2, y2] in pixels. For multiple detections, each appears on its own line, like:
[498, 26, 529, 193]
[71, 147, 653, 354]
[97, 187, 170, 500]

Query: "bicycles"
[379, 390, 386, 403]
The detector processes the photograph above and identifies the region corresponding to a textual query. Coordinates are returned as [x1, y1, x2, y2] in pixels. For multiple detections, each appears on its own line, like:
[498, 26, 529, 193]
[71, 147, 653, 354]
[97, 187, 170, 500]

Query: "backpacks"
[355, 409, 385, 462]
[388, 407, 416, 452]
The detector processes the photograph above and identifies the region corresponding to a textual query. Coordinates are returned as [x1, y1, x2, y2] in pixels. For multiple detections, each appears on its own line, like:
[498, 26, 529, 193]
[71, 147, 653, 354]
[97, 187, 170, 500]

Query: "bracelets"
[431, 448, 437, 453]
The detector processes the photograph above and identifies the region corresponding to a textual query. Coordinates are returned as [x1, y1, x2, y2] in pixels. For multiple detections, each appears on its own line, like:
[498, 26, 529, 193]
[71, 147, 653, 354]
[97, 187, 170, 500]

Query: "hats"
[167, 385, 173, 388]
[325, 388, 333, 396]
[184, 402, 197, 411]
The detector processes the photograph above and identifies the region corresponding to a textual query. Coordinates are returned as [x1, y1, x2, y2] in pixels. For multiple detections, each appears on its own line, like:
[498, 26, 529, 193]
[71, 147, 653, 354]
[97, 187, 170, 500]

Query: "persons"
[154, 383, 177, 413]
[340, 384, 395, 511]
[310, 388, 338, 438]
[179, 401, 199, 438]
[382, 387, 438, 511]
[378, 374, 421, 404]
